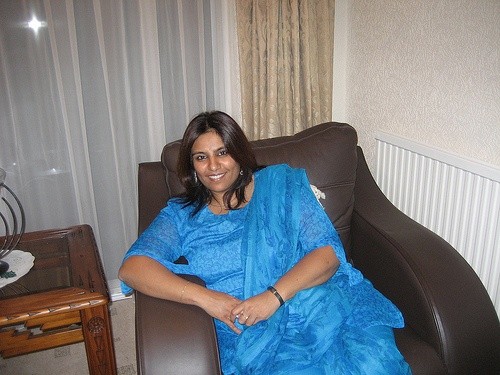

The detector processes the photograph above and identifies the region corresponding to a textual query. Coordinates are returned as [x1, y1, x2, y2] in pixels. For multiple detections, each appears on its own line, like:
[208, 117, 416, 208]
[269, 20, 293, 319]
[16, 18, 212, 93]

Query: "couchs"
[136, 122, 500, 375]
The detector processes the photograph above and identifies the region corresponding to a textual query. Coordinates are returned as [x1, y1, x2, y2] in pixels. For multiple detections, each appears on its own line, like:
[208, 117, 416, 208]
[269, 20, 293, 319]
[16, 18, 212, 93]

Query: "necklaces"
[207, 196, 239, 208]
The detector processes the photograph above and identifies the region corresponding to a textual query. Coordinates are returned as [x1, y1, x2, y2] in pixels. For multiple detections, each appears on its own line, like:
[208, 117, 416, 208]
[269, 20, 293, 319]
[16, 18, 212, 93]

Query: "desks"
[0, 223, 118, 375]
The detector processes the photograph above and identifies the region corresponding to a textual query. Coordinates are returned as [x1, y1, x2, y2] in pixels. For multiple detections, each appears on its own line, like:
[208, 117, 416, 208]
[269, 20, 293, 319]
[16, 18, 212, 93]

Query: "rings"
[235, 315, 239, 319]
[241, 311, 248, 320]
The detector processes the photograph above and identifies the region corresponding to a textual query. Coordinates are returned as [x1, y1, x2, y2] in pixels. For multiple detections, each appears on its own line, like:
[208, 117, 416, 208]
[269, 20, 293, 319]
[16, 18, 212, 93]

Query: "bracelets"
[267, 286, 285, 306]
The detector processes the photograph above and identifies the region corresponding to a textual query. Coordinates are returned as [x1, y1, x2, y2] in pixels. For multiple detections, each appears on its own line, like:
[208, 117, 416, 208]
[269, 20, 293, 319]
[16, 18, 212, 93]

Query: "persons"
[116, 111, 413, 375]
